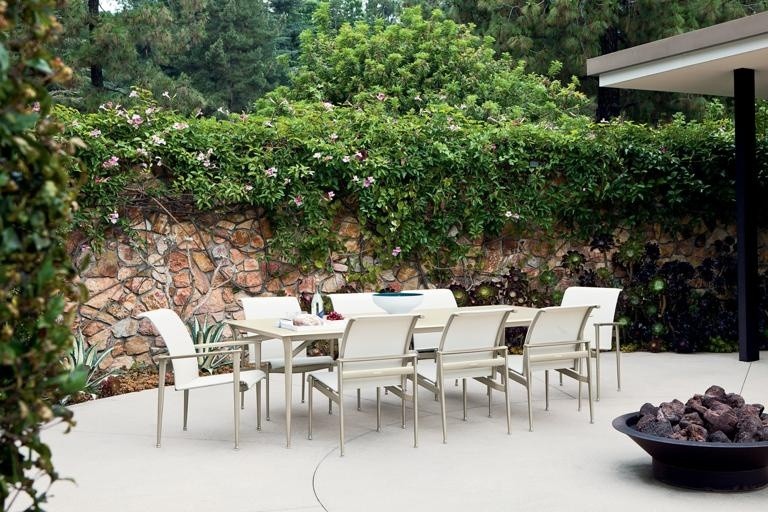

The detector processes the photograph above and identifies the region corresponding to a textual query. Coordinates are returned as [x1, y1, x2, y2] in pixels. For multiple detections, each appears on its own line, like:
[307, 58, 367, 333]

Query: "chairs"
[555, 286, 626, 400]
[401, 307, 519, 444]
[139, 309, 264, 449]
[487, 303, 598, 432]
[237, 295, 334, 420]
[306, 312, 423, 458]
[327, 289, 460, 411]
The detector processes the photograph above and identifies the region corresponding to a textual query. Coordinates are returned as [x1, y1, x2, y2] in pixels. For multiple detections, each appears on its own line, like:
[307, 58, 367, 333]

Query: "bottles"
[310, 278, 325, 320]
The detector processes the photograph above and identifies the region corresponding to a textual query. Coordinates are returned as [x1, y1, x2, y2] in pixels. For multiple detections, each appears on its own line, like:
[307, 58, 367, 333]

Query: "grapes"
[327, 311, 343, 319]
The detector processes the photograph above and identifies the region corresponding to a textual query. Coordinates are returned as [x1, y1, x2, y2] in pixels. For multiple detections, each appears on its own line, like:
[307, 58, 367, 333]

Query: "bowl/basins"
[372, 292, 425, 315]
